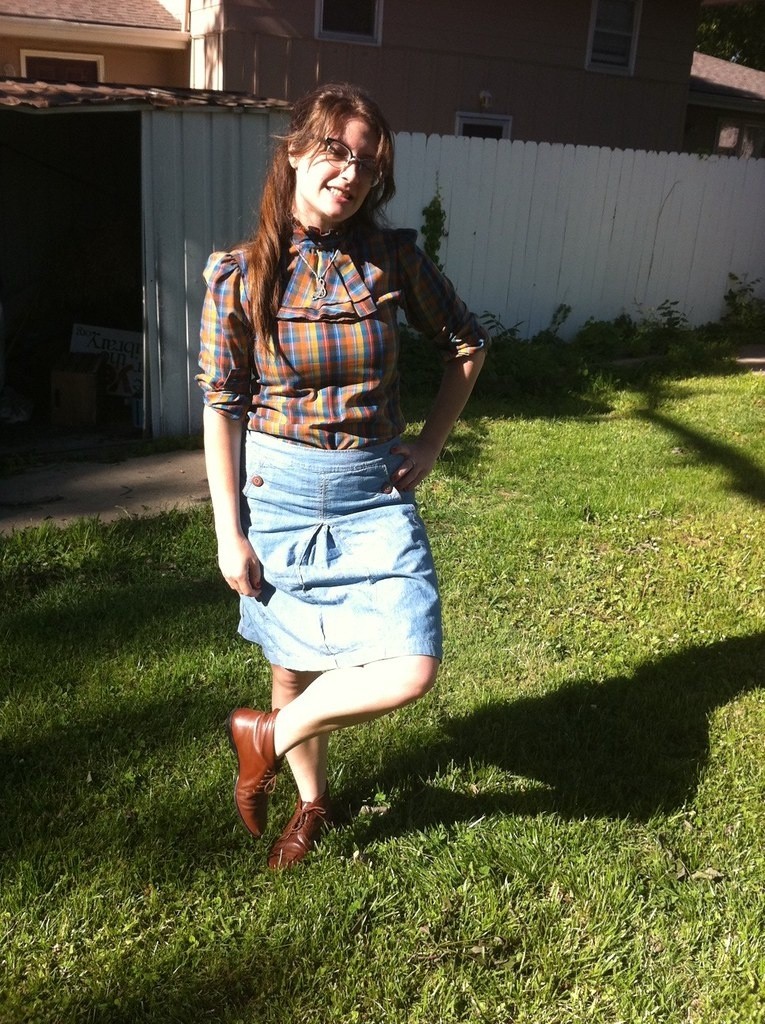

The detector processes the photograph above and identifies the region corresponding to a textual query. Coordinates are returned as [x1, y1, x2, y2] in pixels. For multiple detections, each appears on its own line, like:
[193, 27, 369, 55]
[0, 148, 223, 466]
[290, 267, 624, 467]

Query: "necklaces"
[290, 239, 340, 301]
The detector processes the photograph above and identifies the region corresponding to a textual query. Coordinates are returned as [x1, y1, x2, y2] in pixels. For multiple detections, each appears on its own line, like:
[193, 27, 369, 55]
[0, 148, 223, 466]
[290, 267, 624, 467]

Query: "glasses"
[309, 134, 385, 188]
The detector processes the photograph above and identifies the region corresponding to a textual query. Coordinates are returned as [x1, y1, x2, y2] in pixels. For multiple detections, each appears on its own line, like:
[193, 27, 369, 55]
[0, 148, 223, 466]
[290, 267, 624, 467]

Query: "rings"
[408, 456, 416, 466]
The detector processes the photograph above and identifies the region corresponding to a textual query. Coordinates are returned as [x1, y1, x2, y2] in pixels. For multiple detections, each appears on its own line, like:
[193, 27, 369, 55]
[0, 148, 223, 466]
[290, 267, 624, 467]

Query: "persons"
[193, 83, 491, 872]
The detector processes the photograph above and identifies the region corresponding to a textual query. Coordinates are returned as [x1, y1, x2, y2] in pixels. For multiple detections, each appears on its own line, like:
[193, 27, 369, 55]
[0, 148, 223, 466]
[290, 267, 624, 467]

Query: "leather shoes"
[268, 779, 332, 876]
[226, 707, 285, 837]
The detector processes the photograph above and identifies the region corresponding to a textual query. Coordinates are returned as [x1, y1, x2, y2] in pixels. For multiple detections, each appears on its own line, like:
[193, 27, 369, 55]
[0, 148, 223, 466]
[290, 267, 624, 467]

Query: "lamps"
[479, 90, 493, 109]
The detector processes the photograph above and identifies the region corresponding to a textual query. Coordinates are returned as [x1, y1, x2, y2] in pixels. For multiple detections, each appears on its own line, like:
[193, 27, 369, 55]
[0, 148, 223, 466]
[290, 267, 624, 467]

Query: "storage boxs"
[48, 322, 142, 429]
